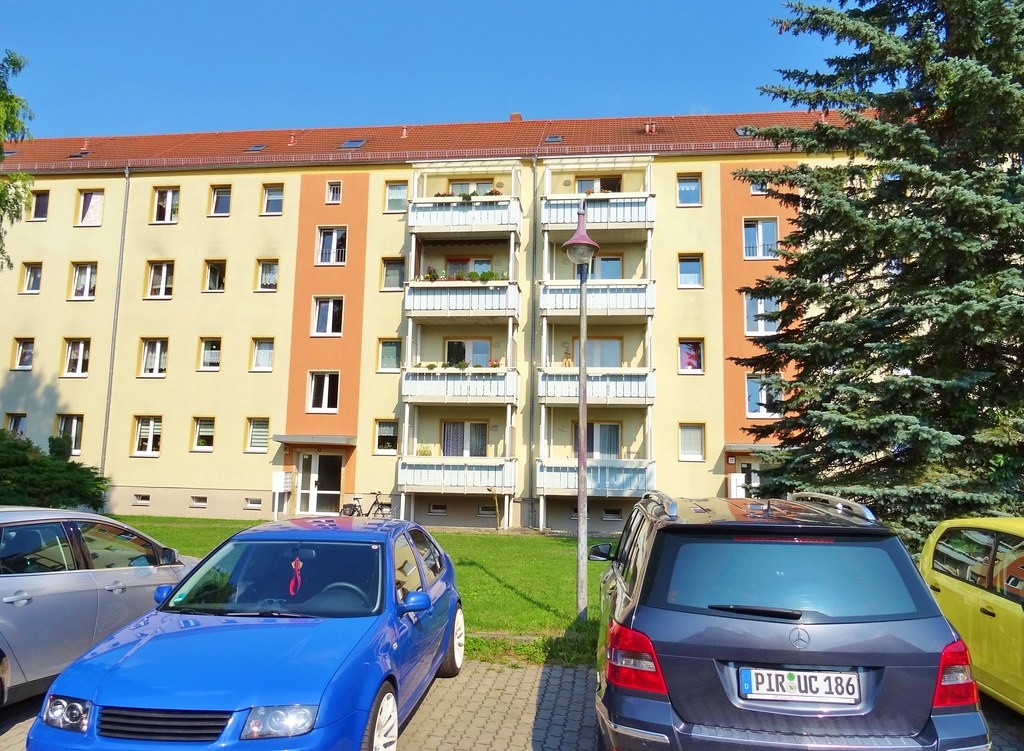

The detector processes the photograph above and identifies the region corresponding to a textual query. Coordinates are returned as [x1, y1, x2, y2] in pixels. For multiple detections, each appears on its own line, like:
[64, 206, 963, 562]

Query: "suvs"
[585, 489, 996, 751]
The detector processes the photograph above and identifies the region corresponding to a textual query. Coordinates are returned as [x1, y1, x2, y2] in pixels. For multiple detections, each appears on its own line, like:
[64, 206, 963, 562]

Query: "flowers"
[433, 191, 455, 197]
[584, 188, 595, 197]
[600, 188, 612, 193]
[488, 358, 500, 368]
[482, 188, 503, 196]
[423, 267, 441, 281]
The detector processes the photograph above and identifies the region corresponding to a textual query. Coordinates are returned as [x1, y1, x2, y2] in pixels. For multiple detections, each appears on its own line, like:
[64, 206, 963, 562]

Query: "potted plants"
[456, 190, 479, 206]
[501, 270, 509, 280]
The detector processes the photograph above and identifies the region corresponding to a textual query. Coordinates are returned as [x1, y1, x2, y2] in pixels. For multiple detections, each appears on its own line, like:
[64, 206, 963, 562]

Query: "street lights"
[562, 198, 599, 632]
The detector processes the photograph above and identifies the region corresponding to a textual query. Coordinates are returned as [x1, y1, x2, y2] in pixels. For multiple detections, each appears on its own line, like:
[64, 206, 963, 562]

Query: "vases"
[433, 202, 450, 206]
[483, 201, 499, 205]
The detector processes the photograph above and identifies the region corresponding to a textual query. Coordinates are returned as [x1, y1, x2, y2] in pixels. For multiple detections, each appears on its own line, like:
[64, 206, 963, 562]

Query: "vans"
[918, 515, 1024, 714]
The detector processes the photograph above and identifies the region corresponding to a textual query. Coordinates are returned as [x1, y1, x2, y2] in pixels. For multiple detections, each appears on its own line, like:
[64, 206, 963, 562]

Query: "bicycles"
[338, 491, 396, 519]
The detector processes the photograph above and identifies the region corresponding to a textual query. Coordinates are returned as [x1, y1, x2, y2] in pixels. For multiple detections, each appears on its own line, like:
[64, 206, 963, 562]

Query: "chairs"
[345, 551, 375, 596]
[0, 531, 51, 572]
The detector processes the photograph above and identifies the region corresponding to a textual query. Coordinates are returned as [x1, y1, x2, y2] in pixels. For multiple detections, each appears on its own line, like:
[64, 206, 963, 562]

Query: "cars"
[24, 515, 465, 751]
[0, 504, 203, 708]
